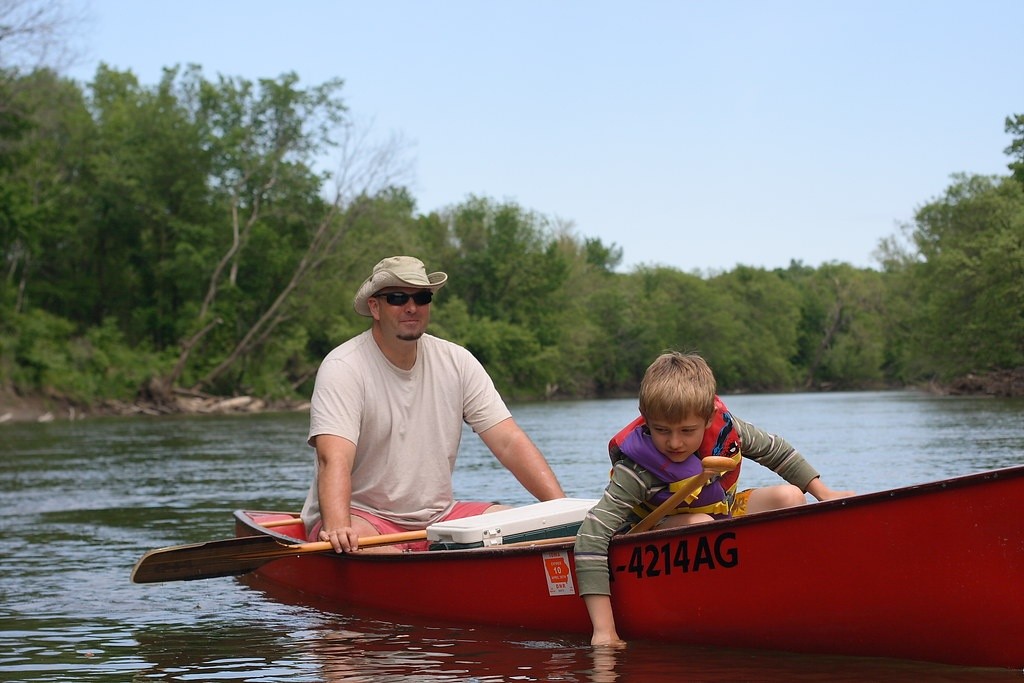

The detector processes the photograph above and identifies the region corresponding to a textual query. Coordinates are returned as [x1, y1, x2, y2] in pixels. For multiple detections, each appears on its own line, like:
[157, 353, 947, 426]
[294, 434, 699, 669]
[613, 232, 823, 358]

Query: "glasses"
[373, 290, 433, 305]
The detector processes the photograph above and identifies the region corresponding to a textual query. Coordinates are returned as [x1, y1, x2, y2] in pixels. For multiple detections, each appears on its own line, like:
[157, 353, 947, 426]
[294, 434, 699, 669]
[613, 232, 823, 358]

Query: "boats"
[230, 466, 1024, 671]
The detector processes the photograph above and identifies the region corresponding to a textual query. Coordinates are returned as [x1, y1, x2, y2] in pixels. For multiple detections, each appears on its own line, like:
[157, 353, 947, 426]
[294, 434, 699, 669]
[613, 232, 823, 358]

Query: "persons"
[574, 354, 859, 645]
[302, 256, 566, 554]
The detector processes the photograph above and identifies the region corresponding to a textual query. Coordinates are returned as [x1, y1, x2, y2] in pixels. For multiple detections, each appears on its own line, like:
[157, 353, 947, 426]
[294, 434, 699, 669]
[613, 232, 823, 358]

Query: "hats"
[353, 255, 447, 316]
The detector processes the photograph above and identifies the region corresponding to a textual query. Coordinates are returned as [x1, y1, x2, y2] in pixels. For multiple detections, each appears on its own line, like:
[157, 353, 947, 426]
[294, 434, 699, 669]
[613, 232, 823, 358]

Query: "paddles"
[623, 455, 738, 537]
[131, 529, 427, 585]
